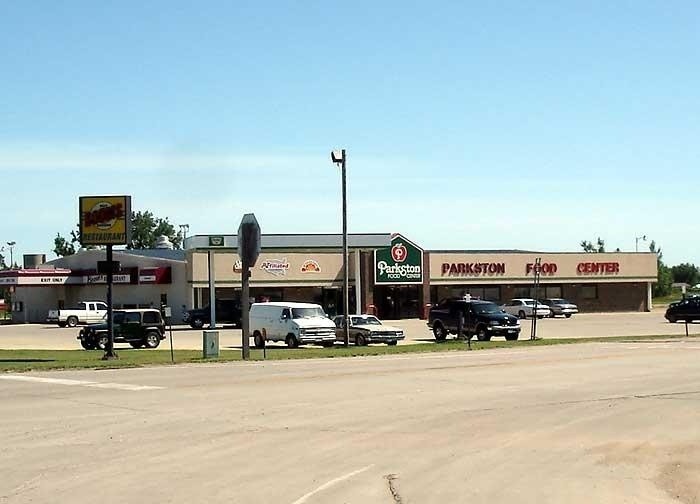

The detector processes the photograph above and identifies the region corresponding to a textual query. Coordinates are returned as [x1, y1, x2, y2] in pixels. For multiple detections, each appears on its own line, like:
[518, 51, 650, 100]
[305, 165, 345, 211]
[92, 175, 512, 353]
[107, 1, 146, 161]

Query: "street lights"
[179, 224, 189, 249]
[7, 241, 16, 269]
[331, 149, 349, 347]
[636, 235, 647, 252]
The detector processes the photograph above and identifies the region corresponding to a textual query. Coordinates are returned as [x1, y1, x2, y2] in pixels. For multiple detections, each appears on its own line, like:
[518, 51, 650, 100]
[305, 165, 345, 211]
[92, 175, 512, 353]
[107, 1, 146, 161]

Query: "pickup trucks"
[427, 299, 521, 341]
[46, 301, 115, 327]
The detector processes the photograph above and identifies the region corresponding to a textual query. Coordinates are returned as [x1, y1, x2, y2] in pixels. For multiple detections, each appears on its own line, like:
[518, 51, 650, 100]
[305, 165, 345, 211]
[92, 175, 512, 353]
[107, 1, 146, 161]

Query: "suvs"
[182, 298, 242, 329]
[77, 308, 166, 350]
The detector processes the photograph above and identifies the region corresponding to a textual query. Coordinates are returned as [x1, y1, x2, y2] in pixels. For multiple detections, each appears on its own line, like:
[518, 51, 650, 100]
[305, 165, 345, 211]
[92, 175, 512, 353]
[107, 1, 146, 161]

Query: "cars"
[332, 314, 405, 346]
[664, 296, 700, 323]
[499, 298, 578, 319]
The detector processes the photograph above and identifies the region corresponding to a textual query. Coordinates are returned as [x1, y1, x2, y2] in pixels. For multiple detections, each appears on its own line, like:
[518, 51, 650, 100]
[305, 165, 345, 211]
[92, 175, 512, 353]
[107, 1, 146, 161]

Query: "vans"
[249, 302, 336, 348]
